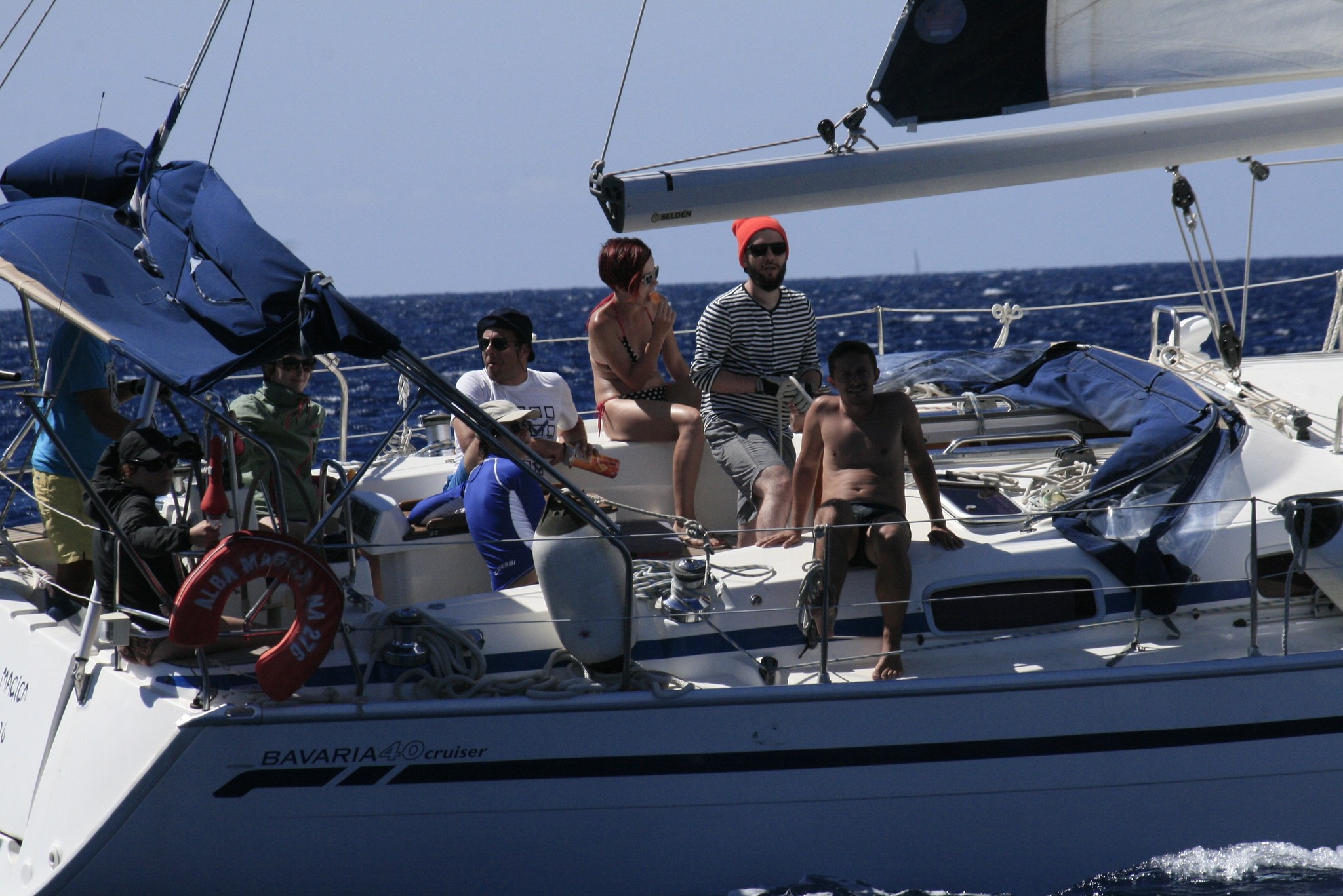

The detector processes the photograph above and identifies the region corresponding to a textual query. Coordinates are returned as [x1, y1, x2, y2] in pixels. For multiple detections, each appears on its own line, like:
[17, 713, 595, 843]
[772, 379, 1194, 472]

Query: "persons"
[689, 216, 824, 549]
[443, 307, 597, 492]
[215, 356, 357, 544]
[585, 237, 729, 549]
[94, 428, 261, 661]
[755, 341, 965, 681]
[30, 321, 172, 601]
[464, 398, 545, 590]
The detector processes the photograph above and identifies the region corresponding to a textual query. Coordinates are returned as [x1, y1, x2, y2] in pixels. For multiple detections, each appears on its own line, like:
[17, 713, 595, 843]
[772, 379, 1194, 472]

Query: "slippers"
[683, 530, 729, 549]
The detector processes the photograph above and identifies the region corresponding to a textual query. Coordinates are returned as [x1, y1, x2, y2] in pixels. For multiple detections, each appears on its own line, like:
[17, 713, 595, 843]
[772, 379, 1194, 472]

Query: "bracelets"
[130, 378, 146, 395]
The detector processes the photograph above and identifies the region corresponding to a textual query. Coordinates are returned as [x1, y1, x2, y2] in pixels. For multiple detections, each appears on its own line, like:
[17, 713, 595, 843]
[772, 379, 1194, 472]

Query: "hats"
[479, 400, 541, 423]
[732, 215, 789, 268]
[119, 428, 174, 463]
[477, 308, 535, 362]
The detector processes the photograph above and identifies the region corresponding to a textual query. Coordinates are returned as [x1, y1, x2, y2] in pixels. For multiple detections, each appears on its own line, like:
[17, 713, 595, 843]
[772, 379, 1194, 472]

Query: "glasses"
[479, 337, 517, 351]
[748, 241, 787, 256]
[509, 420, 534, 435]
[276, 356, 317, 372]
[641, 266, 659, 286]
[143, 455, 177, 473]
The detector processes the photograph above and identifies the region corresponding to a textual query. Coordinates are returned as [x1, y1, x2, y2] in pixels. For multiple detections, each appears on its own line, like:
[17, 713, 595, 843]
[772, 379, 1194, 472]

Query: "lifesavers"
[168, 530, 346, 703]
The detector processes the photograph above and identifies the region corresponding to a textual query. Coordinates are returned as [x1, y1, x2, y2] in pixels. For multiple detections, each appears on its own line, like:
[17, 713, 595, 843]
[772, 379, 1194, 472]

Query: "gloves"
[754, 375, 798, 403]
[793, 380, 816, 414]
[169, 431, 205, 462]
[130, 377, 172, 402]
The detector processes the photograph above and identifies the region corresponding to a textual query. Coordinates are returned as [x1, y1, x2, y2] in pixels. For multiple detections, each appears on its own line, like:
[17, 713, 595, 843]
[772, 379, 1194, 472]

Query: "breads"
[346, 469, 356, 482]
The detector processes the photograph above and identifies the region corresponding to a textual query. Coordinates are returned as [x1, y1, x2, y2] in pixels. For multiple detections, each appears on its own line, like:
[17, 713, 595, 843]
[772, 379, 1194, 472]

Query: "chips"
[650, 291, 661, 304]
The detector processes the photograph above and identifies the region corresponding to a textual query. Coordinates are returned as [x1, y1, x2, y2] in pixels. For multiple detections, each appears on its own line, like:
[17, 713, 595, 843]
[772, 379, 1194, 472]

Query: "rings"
[950, 536, 953, 539]
